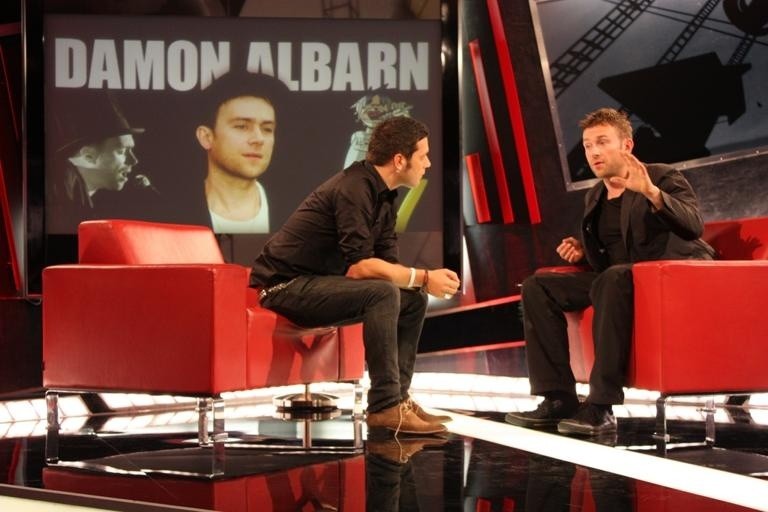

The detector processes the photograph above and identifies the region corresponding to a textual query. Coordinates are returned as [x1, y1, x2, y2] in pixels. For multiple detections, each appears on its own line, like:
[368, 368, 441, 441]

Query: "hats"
[194, 69, 295, 116]
[54, 102, 144, 155]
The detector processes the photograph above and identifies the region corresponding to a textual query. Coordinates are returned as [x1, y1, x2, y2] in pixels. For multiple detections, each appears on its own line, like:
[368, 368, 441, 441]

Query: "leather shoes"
[404, 398, 452, 424]
[366, 399, 446, 435]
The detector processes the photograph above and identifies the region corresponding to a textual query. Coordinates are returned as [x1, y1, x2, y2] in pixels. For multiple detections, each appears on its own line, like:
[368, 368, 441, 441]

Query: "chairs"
[535, 217, 768, 455]
[43, 219, 366, 481]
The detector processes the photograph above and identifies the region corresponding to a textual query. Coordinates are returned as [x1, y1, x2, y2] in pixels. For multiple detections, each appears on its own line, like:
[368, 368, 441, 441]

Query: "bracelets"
[406, 266, 416, 289]
[422, 267, 428, 290]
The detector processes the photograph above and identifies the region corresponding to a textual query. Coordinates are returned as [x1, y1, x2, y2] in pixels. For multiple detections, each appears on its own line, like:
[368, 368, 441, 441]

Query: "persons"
[505, 106, 718, 436]
[43, 100, 145, 233]
[152, 70, 299, 233]
[249, 116, 461, 434]
[362, 435, 448, 511]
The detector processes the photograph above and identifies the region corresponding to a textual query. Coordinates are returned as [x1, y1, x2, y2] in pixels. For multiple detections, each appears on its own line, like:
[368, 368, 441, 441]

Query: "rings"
[444, 294, 452, 299]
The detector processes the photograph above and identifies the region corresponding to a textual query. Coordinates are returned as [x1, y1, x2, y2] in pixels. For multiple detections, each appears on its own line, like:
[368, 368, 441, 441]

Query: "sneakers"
[558, 406, 618, 436]
[505, 397, 583, 426]
